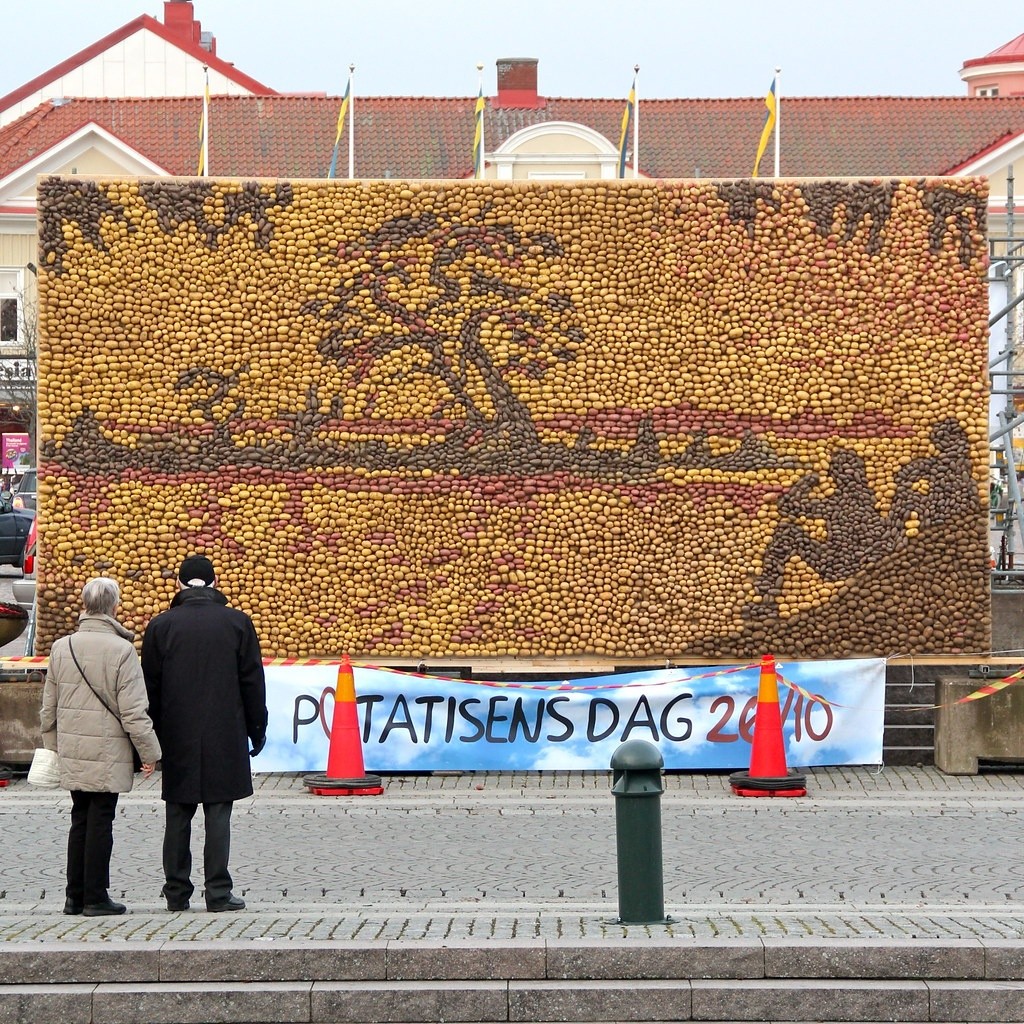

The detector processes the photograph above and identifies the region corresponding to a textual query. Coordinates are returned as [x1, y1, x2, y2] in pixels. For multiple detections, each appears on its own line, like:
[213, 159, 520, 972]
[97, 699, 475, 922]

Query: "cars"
[0, 470, 36, 612]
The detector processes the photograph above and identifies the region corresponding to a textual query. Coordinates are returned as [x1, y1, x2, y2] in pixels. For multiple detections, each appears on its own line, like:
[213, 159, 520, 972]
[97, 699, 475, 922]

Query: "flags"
[472, 82, 487, 181]
[752, 79, 777, 178]
[197, 76, 210, 179]
[618, 79, 641, 179]
[328, 81, 351, 179]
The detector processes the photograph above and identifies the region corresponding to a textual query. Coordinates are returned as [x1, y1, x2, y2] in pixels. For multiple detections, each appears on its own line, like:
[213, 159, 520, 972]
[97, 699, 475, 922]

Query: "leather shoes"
[63, 895, 82, 915]
[169, 899, 190, 911]
[208, 893, 247, 914]
[82, 899, 127, 918]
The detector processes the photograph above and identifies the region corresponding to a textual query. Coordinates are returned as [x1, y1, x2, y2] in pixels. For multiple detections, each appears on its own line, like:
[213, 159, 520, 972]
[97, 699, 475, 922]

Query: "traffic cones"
[304, 655, 396, 802]
[726, 654, 811, 799]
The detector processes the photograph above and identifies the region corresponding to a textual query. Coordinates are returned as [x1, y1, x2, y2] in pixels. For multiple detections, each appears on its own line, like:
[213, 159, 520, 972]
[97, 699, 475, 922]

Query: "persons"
[39, 577, 162, 917]
[141, 555, 268, 912]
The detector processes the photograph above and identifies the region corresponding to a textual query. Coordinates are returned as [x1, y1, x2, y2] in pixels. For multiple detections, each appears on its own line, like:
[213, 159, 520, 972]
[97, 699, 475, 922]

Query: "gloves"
[249, 724, 267, 758]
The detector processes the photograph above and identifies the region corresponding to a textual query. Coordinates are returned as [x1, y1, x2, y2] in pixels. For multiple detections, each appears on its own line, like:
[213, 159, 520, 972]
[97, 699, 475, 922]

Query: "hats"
[178, 555, 216, 589]
